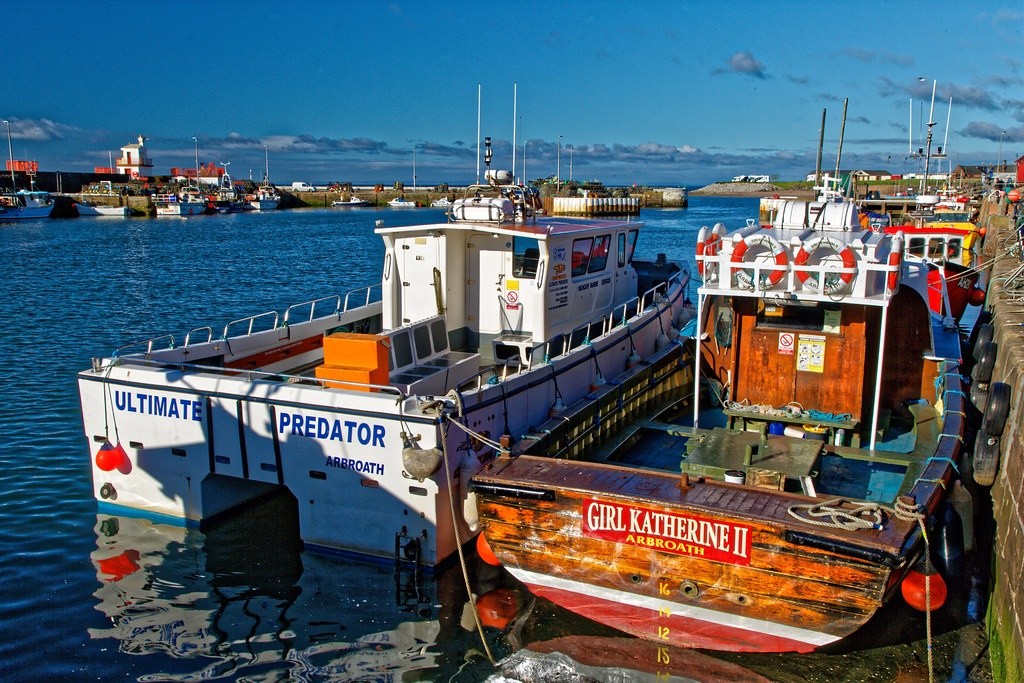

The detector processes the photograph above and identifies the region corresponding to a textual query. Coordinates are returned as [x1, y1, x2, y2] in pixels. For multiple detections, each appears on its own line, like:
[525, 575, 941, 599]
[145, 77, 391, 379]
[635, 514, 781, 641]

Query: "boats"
[811, 93, 982, 267]
[387, 197, 417, 207]
[468, 88, 975, 654]
[0, 187, 75, 220]
[214, 198, 245, 214]
[77, 72, 699, 576]
[75, 201, 131, 216]
[331, 197, 369, 208]
[154, 193, 207, 215]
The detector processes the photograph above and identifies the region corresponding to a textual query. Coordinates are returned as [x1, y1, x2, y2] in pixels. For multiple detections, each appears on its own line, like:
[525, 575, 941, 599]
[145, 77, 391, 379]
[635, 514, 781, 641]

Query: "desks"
[680, 426, 824, 497]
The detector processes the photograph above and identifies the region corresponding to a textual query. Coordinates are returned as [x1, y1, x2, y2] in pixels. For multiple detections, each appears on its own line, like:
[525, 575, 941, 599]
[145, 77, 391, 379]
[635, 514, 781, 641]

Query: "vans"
[292, 181, 316, 192]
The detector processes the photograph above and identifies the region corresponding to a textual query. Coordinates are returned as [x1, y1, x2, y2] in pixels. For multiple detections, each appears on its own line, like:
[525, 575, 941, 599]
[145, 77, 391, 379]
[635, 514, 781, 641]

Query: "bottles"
[769, 421, 783, 435]
[850, 433, 860, 449]
[834, 428, 845, 447]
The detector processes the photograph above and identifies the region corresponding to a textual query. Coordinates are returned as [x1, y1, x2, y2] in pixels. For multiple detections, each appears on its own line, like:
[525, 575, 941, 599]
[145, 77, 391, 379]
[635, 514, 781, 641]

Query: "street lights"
[557, 135, 563, 192]
[412, 146, 416, 193]
[264, 144, 269, 179]
[3, 120, 17, 191]
[523, 141, 527, 187]
[192, 136, 199, 189]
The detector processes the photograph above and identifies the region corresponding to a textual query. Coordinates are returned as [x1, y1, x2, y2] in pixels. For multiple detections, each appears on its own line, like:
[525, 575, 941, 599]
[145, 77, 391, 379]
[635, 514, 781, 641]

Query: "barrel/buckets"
[723, 470, 745, 484]
[803, 424, 828, 440]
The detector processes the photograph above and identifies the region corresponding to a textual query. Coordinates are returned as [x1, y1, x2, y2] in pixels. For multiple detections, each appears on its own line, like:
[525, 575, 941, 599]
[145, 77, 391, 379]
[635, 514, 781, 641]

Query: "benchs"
[573, 381, 709, 463]
[892, 398, 941, 510]
[603, 462, 892, 508]
[492, 334, 533, 367]
[723, 408, 859, 445]
[376, 315, 480, 397]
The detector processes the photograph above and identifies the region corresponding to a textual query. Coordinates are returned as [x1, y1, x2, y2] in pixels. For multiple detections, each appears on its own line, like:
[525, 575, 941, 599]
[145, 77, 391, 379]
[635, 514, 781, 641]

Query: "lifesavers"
[730, 233, 787, 291]
[966, 324, 1010, 487]
[712, 222, 727, 256]
[695, 226, 713, 276]
[885, 236, 904, 297]
[795, 235, 856, 294]
